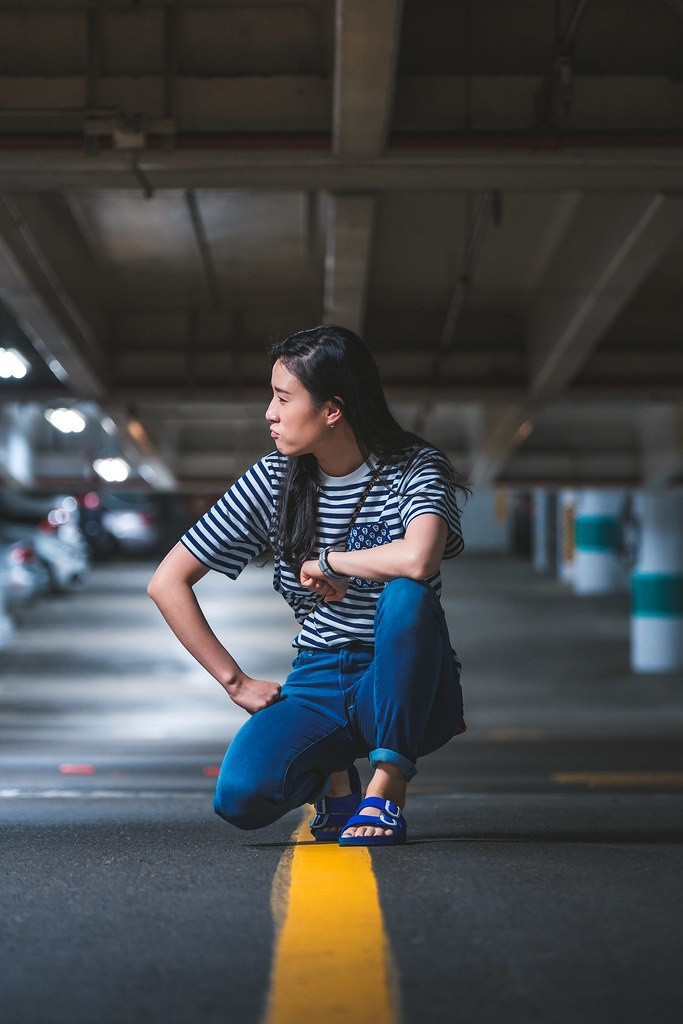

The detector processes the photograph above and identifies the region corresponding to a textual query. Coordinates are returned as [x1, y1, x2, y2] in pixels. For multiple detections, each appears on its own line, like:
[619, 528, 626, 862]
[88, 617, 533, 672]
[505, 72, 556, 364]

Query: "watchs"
[319, 546, 352, 580]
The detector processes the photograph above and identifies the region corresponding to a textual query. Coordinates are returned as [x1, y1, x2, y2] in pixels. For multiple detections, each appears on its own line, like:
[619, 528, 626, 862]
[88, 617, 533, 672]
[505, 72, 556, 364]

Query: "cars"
[0, 386, 193, 650]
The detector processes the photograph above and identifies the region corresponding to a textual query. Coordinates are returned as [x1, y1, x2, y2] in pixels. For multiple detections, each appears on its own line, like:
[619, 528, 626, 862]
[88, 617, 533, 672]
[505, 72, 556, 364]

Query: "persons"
[148, 322, 475, 847]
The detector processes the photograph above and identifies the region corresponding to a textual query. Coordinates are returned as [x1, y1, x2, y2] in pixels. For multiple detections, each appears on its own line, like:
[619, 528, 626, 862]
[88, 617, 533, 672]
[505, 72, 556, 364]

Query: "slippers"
[309, 765, 362, 841]
[339, 796, 408, 847]
